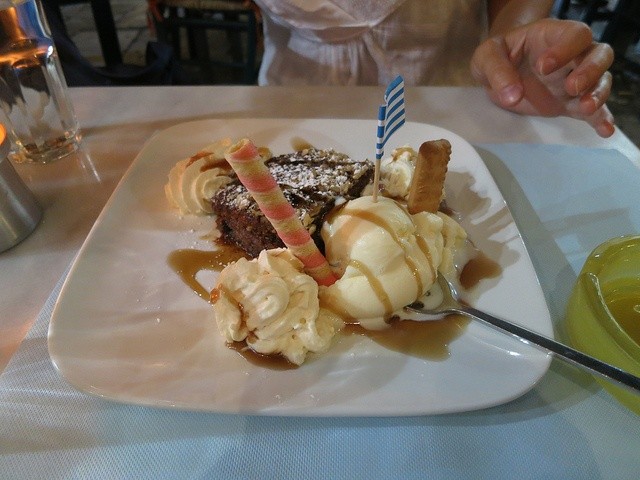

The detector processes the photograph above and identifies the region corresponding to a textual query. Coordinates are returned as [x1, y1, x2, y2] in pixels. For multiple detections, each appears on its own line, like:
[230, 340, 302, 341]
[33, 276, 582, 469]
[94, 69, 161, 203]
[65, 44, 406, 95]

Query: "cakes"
[205, 145, 374, 264]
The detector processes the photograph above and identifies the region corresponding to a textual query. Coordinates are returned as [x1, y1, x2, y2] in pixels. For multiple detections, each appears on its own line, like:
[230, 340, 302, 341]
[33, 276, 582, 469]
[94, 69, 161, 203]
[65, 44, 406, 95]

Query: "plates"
[45, 114, 557, 422]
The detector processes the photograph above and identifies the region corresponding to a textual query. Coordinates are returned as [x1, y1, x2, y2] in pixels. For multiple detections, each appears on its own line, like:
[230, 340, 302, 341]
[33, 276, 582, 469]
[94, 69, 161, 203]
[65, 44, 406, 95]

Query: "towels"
[1, 142, 640, 479]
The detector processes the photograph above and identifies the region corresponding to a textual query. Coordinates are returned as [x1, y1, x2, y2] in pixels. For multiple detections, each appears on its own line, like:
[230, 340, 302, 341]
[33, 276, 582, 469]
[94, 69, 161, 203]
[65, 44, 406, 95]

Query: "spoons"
[391, 265, 640, 395]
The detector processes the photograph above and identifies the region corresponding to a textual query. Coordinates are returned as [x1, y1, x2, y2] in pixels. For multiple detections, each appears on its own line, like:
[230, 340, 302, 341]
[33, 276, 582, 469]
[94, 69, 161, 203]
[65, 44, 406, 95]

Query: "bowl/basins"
[565, 233, 640, 420]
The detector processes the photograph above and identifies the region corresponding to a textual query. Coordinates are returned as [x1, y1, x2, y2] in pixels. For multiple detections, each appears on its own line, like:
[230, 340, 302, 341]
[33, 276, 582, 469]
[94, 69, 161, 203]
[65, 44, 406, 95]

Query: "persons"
[251, 0, 615, 138]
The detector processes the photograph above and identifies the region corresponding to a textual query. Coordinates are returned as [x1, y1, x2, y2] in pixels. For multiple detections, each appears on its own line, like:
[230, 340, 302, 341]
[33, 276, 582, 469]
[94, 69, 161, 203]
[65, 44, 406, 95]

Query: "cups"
[0, 152, 47, 255]
[0, 0, 84, 166]
